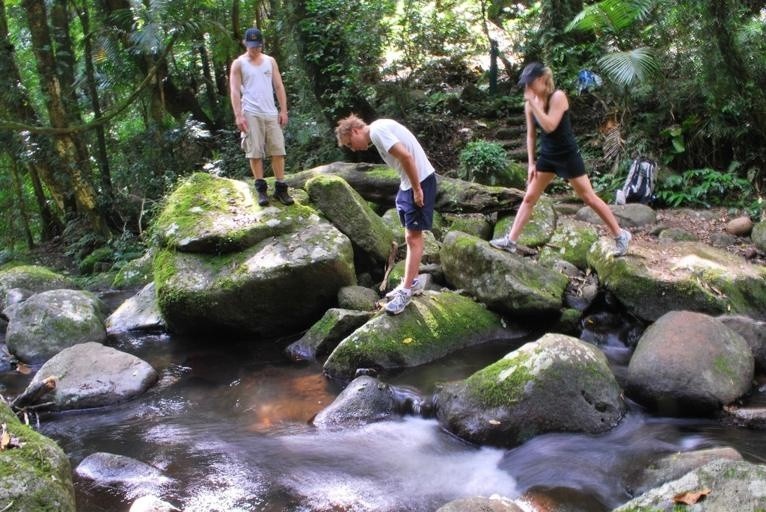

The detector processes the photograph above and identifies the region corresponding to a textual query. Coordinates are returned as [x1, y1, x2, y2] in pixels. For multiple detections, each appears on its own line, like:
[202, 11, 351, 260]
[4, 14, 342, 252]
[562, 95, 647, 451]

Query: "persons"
[331, 110, 436, 315]
[229, 27, 295, 207]
[487, 61, 632, 258]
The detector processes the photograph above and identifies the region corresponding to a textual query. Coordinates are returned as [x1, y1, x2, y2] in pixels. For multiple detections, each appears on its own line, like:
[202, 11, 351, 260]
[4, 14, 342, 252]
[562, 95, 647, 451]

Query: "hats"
[517, 63, 543, 87]
[245, 28, 263, 49]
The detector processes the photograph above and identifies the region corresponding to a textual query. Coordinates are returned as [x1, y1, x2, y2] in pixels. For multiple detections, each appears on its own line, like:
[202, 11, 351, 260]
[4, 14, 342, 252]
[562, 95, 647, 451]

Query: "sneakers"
[385, 276, 423, 300]
[386, 289, 412, 315]
[614, 229, 632, 256]
[489, 233, 517, 254]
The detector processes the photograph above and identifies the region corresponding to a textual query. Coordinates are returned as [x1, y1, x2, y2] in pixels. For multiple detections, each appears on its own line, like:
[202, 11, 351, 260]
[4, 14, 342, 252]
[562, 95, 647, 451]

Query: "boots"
[273, 182, 294, 204]
[255, 180, 269, 206]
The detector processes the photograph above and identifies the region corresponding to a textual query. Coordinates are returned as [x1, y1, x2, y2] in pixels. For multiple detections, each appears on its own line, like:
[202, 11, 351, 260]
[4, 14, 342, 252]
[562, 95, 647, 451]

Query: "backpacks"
[615, 158, 658, 209]
